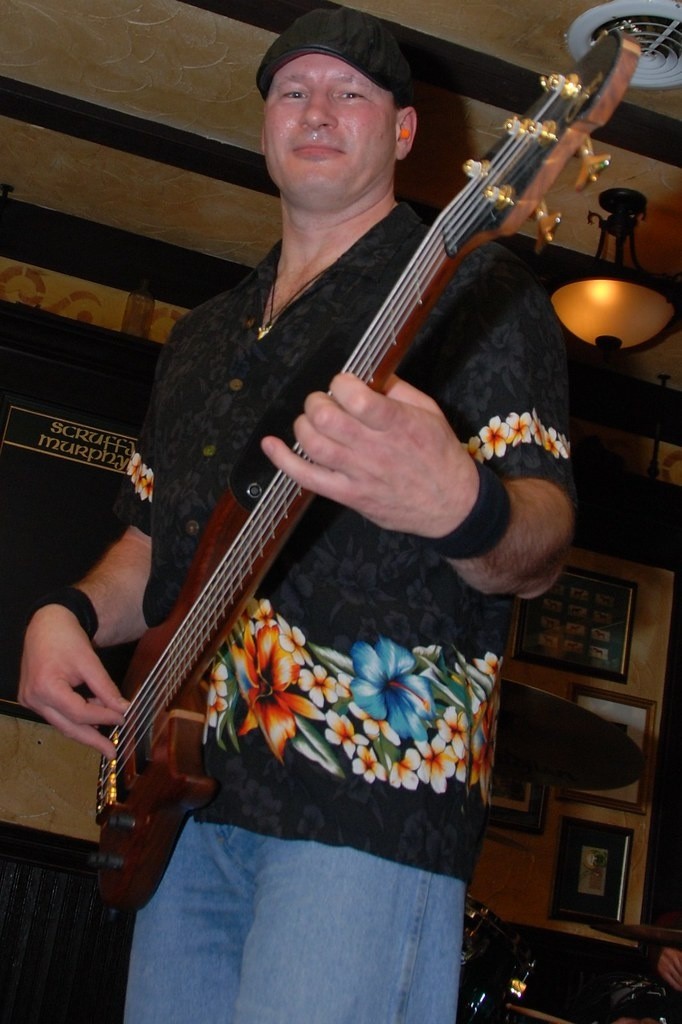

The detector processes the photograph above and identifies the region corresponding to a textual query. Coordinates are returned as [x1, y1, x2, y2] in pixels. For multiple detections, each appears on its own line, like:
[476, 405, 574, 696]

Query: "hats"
[255, 7, 412, 102]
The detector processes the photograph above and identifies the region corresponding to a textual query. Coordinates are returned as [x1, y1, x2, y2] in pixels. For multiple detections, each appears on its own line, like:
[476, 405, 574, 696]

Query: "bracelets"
[427, 457, 512, 560]
[22, 587, 98, 642]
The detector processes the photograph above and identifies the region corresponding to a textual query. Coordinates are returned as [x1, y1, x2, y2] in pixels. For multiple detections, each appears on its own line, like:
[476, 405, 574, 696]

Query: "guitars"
[90, 25, 643, 916]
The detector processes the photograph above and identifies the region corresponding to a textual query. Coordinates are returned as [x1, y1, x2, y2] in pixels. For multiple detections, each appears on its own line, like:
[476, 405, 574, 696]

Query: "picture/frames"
[511, 563, 638, 684]
[549, 816, 638, 926]
[485, 780, 551, 834]
[556, 682, 658, 814]
[0, 301, 178, 727]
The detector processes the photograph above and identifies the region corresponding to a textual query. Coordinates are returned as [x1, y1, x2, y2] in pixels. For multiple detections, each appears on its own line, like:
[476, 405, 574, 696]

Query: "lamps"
[551, 187, 682, 358]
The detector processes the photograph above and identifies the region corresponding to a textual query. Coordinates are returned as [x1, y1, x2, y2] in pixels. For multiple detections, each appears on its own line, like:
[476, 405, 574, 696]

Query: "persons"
[16, 7, 579, 1024]
[610, 910, 682, 1024]
